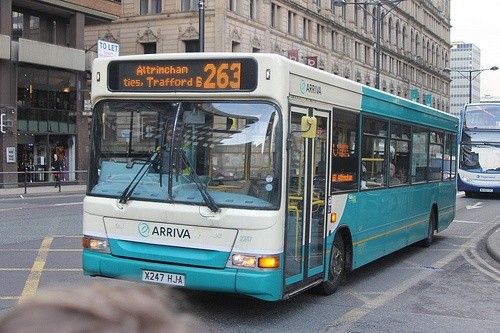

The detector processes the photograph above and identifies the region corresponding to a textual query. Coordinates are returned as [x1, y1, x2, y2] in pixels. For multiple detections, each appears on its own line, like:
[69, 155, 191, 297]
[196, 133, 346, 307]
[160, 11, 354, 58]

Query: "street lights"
[334, 0, 405, 89]
[443, 66, 499, 104]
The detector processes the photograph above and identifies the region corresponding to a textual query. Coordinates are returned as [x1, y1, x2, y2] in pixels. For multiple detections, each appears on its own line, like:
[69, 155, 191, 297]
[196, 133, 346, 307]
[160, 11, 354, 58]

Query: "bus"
[81, 51, 459, 302]
[457, 100, 500, 198]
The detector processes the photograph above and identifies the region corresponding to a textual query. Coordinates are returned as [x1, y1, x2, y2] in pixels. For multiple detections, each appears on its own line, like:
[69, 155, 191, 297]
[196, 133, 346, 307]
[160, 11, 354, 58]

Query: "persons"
[51, 153, 60, 188]
[0, 279, 210, 333]
[389, 162, 400, 185]
[152, 122, 192, 176]
